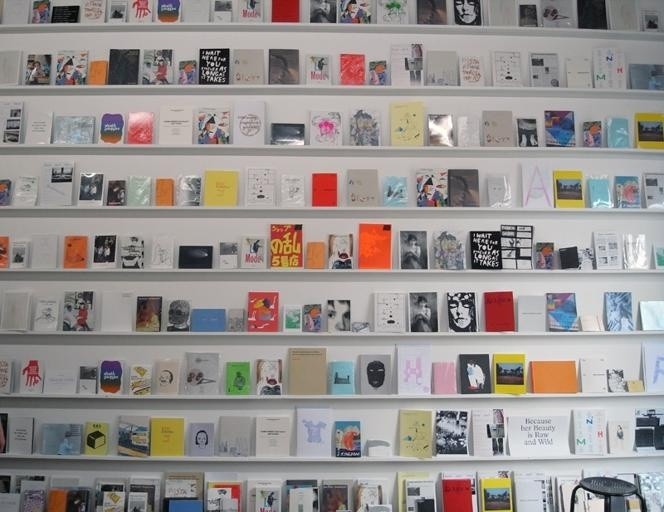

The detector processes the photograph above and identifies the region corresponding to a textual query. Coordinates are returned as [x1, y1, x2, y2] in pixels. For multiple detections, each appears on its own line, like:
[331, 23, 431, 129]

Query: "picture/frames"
[398, 230, 431, 269]
[459, 354, 492, 394]
[240, 236, 268, 269]
[283, 305, 302, 332]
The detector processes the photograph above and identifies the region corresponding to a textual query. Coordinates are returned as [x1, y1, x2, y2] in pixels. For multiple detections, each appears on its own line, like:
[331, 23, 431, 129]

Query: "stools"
[569, 476, 648, 512]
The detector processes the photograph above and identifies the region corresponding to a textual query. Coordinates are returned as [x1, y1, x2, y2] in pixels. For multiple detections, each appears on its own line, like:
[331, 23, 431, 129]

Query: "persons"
[401, 233, 422, 269]
[413, 296, 433, 332]
[155, 59, 169, 84]
[454, 0, 479, 25]
[326, 299, 352, 332]
[446, 292, 478, 332]
[143, 62, 155, 84]
[64, 303, 77, 332]
[77, 302, 90, 331]
[198, 116, 226, 145]
[29, 61, 46, 82]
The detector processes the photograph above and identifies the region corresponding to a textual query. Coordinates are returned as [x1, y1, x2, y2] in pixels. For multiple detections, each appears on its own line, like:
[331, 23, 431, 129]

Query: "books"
[0, 99, 96, 146]
[579, 290, 663, 332]
[512, 468, 664, 512]
[232, 47, 302, 86]
[225, 346, 393, 397]
[0, 411, 110, 457]
[233, 98, 383, 147]
[63, 290, 95, 331]
[0, 233, 89, 270]
[238, 0, 263, 24]
[592, 47, 626, 89]
[100, 289, 245, 333]
[55, 48, 141, 86]
[460, 52, 485, 86]
[483, 290, 580, 334]
[491, 51, 522, 87]
[0, 159, 76, 208]
[396, 470, 439, 512]
[202, 166, 339, 208]
[128, 351, 220, 396]
[439, 470, 514, 512]
[0, 355, 125, 396]
[471, 406, 664, 458]
[486, 173, 513, 208]
[218, 223, 393, 271]
[628, 63, 664, 91]
[51, 5, 80, 23]
[285, 478, 393, 512]
[543, 110, 664, 150]
[128, 0, 153, 23]
[528, 340, 664, 394]
[25, 53, 52, 85]
[566, 55, 593, 89]
[557, 229, 664, 271]
[510, 1, 664, 34]
[156, 0, 181, 23]
[163, 471, 283, 512]
[0, 469, 92, 512]
[417, 0, 448, 25]
[528, 50, 562, 88]
[207, 0, 232, 23]
[389, 39, 425, 87]
[376, 0, 409, 24]
[117, 414, 291, 459]
[309, 0, 337, 24]
[305, 51, 388, 87]
[246, 290, 322, 334]
[271, 0, 300, 23]
[395, 345, 526, 395]
[1, 289, 60, 332]
[83, 0, 107, 24]
[177, 45, 231, 86]
[91, 234, 214, 269]
[27, 0, 51, 26]
[426, 50, 459, 86]
[374, 290, 406, 333]
[397, 223, 555, 270]
[196, 106, 230, 145]
[294, 406, 471, 458]
[93, 474, 161, 512]
[98, 104, 194, 146]
[142, 48, 173, 85]
[520, 165, 664, 209]
[345, 167, 481, 208]
[389, 100, 539, 148]
[78, 171, 202, 208]
[107, 0, 128, 24]
[338, 0, 372, 24]
